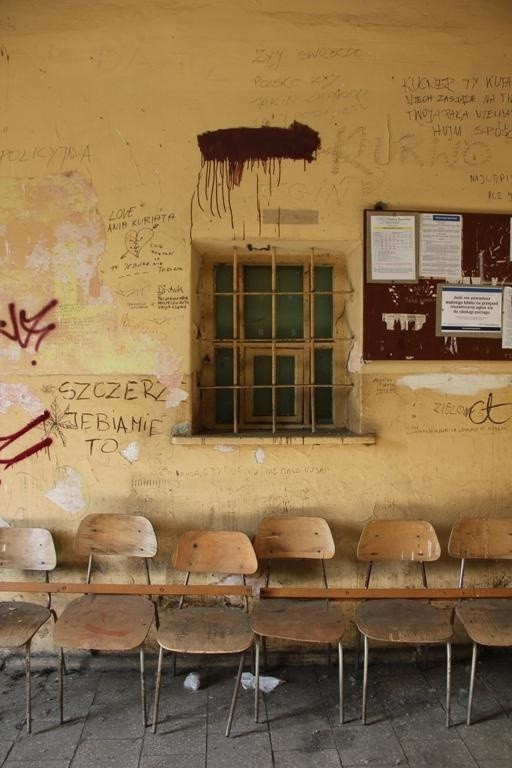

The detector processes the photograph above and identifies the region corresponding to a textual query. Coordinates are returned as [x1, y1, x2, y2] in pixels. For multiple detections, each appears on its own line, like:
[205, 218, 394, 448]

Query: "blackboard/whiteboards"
[354, 207, 512, 375]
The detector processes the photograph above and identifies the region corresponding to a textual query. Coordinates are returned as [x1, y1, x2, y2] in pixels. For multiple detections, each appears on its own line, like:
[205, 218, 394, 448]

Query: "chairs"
[0, 527, 67, 732]
[53, 514, 159, 728]
[448, 517, 512, 725]
[152, 530, 259, 734]
[251, 517, 347, 725]
[356, 520, 454, 727]
